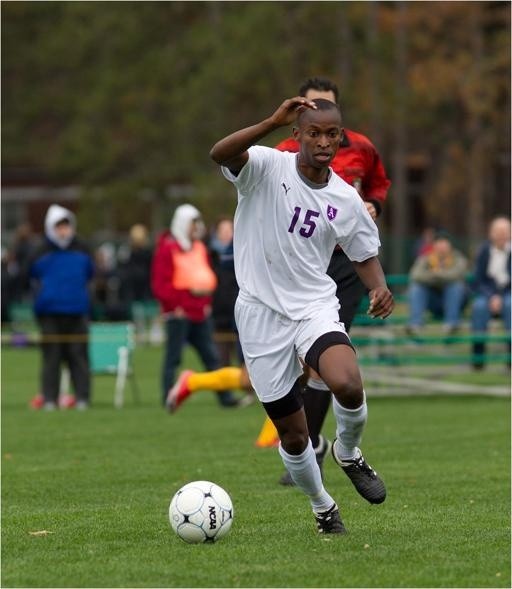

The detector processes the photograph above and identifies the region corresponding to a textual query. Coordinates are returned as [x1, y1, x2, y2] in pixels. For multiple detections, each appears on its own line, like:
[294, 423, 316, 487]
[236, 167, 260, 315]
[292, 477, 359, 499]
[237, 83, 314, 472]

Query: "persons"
[209, 96, 395, 535]
[152, 201, 256, 412]
[25, 204, 96, 412]
[468, 215, 511, 370]
[275, 77, 391, 487]
[405, 232, 471, 340]
[0, 203, 235, 344]
[160, 359, 291, 450]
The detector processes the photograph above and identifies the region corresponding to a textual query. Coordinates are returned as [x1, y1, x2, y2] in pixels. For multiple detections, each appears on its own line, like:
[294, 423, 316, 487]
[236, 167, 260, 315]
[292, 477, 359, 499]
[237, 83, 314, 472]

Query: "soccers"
[169, 481, 233, 543]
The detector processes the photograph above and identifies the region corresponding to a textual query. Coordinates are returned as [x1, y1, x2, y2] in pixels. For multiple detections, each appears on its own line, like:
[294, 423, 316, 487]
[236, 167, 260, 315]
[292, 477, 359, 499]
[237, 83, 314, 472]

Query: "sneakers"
[313, 503, 346, 535]
[256, 434, 333, 485]
[332, 438, 385, 504]
[167, 370, 192, 415]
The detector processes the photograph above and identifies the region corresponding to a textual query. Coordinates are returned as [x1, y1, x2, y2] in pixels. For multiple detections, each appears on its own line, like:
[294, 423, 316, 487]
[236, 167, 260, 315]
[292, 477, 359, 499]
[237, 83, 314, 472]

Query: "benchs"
[348, 271, 511, 367]
